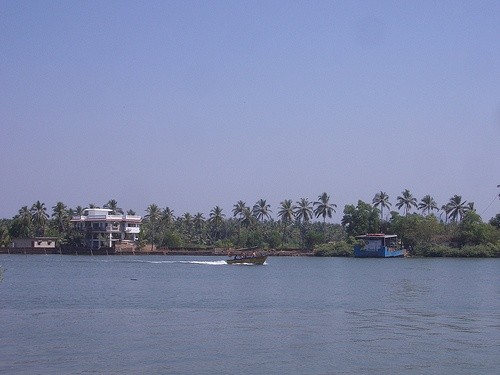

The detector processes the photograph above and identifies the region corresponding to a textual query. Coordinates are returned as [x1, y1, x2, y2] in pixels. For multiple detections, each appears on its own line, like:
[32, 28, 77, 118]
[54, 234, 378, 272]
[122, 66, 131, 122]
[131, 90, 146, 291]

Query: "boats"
[225, 255, 267, 266]
[354, 233, 406, 258]
